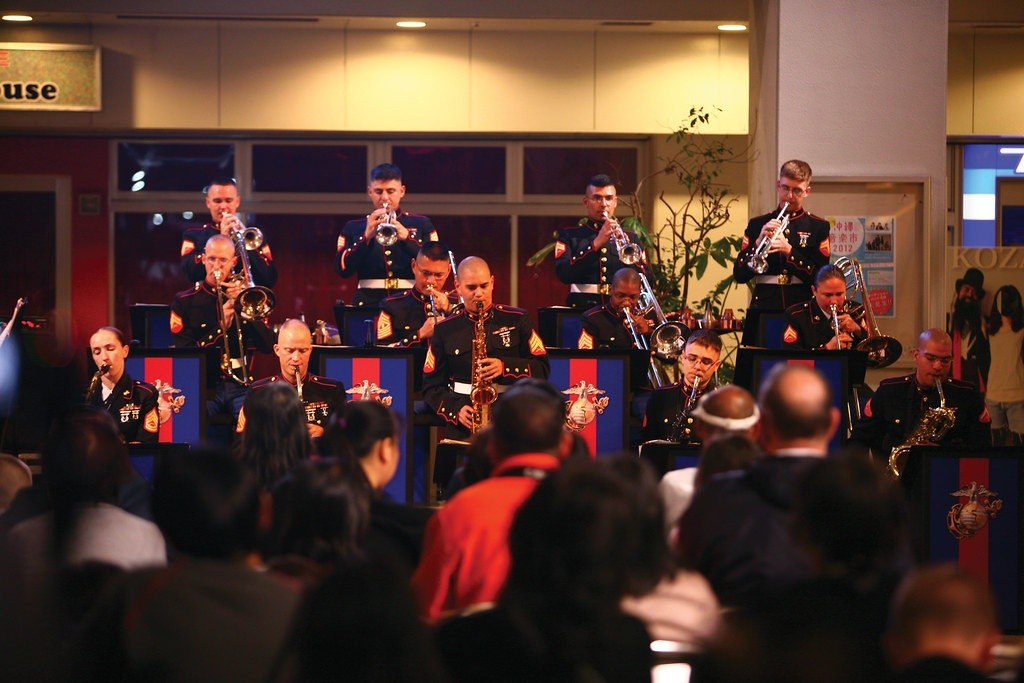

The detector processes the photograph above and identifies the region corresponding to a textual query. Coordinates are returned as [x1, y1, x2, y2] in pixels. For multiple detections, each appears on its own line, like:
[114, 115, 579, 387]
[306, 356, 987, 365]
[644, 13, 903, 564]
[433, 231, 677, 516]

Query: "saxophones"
[666, 375, 701, 443]
[469, 301, 498, 436]
[884, 375, 959, 486]
[296, 367, 304, 403]
[85, 363, 110, 403]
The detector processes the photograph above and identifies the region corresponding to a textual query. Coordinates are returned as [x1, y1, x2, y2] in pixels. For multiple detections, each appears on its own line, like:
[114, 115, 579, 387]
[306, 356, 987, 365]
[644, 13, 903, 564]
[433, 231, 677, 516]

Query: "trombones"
[622, 273, 691, 388]
[425, 251, 465, 316]
[830, 255, 904, 465]
[213, 231, 277, 387]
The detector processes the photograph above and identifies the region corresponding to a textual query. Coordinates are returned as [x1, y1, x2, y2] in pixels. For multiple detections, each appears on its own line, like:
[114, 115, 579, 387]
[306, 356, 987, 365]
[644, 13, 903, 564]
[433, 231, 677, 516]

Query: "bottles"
[679, 300, 741, 332]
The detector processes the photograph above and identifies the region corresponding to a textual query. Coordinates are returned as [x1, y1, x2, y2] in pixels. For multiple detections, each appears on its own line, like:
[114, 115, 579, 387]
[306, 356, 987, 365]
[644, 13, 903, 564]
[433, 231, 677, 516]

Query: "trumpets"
[375, 204, 398, 246]
[746, 201, 793, 273]
[603, 210, 642, 265]
[221, 212, 263, 250]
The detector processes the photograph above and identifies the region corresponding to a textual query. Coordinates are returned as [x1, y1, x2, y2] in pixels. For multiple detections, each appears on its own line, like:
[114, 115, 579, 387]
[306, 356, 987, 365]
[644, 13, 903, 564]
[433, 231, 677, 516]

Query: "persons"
[861, 328, 991, 468]
[732, 160, 831, 398]
[246, 319, 346, 439]
[946, 267, 990, 389]
[576, 267, 654, 352]
[783, 264, 873, 352]
[335, 163, 440, 306]
[371, 241, 455, 347]
[421, 255, 551, 494]
[0, 363, 1000, 683]
[169, 234, 278, 423]
[176, 175, 279, 291]
[984, 285, 1024, 446]
[555, 173, 655, 317]
[645, 329, 721, 457]
[87, 326, 159, 444]
